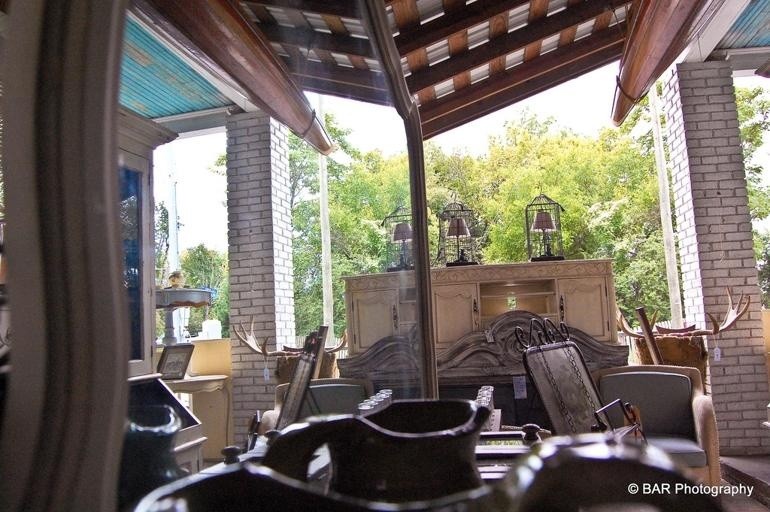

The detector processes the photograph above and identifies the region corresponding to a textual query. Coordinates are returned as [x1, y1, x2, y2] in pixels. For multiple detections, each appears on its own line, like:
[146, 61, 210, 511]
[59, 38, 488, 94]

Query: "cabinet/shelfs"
[479, 262, 560, 331]
[345, 269, 398, 361]
[432, 261, 482, 356]
[398, 270, 418, 337]
[561, 258, 617, 346]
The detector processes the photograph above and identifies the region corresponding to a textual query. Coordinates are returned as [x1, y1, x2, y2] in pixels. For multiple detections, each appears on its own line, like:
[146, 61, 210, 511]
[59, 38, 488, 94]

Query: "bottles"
[475, 384, 497, 431]
[357, 387, 393, 416]
[169, 270, 185, 288]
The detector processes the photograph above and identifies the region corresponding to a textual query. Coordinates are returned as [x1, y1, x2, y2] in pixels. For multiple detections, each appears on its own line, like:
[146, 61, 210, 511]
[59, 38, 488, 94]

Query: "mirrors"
[5, 1, 439, 512]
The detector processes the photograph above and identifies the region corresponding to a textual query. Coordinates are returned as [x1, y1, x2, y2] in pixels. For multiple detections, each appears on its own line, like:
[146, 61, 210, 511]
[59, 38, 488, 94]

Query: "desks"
[161, 373, 233, 453]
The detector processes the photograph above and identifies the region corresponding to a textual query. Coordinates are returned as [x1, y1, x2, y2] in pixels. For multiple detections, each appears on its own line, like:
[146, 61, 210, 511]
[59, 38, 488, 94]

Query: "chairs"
[235, 394, 721, 512]
[249, 376, 375, 455]
[587, 366, 723, 502]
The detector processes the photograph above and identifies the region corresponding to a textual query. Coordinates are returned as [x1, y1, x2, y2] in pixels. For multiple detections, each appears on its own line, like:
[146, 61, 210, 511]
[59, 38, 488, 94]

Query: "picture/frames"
[155, 342, 195, 381]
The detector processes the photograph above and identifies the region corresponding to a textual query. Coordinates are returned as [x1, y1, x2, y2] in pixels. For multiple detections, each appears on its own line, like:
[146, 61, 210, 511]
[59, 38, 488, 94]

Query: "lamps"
[528, 209, 558, 256]
[391, 220, 415, 266]
[442, 216, 475, 263]
